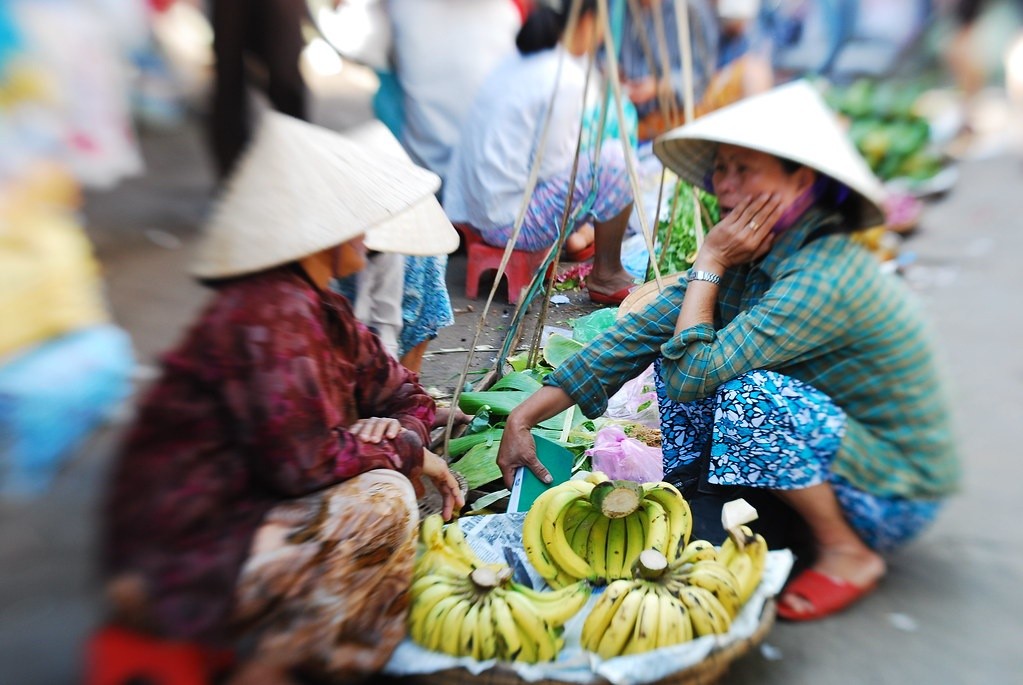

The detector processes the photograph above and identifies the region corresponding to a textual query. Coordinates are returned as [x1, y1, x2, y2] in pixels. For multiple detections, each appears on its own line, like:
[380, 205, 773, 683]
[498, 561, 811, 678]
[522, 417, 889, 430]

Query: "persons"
[195, 1, 537, 383]
[495, 79, 961, 621]
[910, 0, 1023, 156]
[438, 0, 645, 303]
[88, 114, 465, 685]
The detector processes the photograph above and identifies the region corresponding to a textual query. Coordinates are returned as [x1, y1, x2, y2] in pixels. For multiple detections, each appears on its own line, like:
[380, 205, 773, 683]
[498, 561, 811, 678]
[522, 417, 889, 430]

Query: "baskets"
[386, 601, 776, 685]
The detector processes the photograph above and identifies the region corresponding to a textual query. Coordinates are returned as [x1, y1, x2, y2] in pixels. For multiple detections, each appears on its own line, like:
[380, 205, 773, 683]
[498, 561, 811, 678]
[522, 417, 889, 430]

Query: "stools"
[448, 223, 555, 306]
[84, 620, 237, 685]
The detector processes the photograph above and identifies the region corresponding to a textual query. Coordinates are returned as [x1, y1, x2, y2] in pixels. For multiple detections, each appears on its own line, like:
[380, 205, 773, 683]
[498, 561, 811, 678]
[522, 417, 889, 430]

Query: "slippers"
[589, 285, 639, 304]
[778, 568, 874, 620]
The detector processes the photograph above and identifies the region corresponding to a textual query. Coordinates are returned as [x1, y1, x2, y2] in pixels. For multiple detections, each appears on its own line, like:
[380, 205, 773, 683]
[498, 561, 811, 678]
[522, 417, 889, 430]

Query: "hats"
[185, 111, 440, 277]
[651, 78, 890, 231]
[335, 118, 461, 256]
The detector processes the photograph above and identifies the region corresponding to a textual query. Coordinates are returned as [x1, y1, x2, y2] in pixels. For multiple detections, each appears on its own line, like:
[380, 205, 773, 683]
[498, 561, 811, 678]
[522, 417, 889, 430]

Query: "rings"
[749, 222, 759, 231]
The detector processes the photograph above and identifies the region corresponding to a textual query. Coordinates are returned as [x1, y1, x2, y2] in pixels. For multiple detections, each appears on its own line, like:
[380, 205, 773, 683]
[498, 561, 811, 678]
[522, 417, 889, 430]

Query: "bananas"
[409, 472, 768, 664]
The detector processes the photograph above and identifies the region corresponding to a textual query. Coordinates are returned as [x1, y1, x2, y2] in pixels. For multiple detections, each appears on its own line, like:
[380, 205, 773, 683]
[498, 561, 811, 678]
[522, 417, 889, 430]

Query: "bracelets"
[686, 268, 721, 285]
